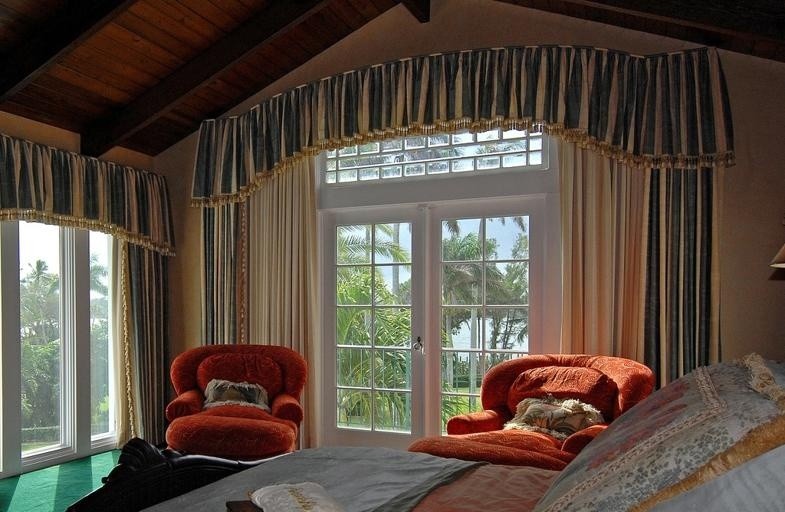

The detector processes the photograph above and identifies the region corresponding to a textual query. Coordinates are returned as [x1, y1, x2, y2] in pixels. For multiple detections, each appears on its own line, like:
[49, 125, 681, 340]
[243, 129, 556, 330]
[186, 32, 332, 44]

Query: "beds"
[63, 349, 785, 512]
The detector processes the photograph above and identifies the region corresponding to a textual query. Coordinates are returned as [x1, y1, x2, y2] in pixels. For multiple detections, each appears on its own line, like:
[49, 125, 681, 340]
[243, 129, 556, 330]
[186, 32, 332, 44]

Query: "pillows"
[504, 397, 600, 443]
[202, 378, 270, 412]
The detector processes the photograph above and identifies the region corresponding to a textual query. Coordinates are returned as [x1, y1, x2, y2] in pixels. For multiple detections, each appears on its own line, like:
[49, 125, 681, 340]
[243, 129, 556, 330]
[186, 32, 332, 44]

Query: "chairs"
[405, 353, 655, 472]
[164, 344, 308, 461]
